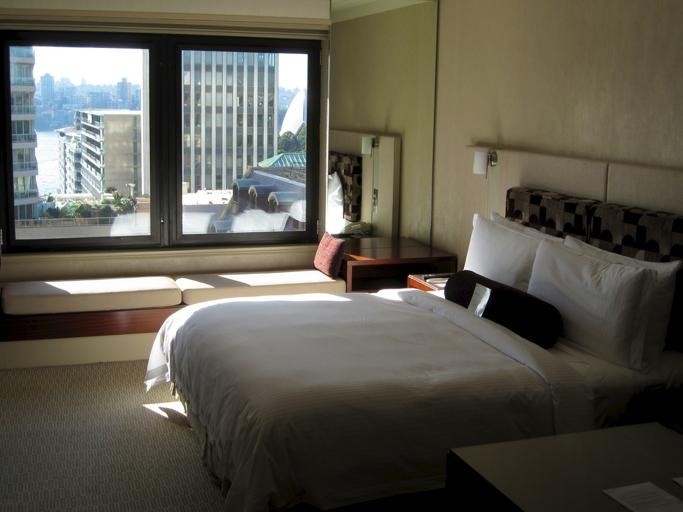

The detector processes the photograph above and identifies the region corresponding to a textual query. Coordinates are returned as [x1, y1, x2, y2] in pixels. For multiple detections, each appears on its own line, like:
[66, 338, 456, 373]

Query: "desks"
[346, 234, 460, 293]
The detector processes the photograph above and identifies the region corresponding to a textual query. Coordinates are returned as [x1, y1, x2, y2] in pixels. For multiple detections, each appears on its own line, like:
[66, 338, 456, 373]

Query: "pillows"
[443, 209, 679, 371]
[312, 231, 347, 277]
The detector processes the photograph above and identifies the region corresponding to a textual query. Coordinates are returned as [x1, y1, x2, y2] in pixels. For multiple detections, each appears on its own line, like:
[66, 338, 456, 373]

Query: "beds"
[150, 182, 680, 510]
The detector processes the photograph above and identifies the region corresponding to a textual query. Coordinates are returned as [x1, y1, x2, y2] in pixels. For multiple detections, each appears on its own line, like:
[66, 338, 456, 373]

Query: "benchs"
[9, 270, 347, 374]
